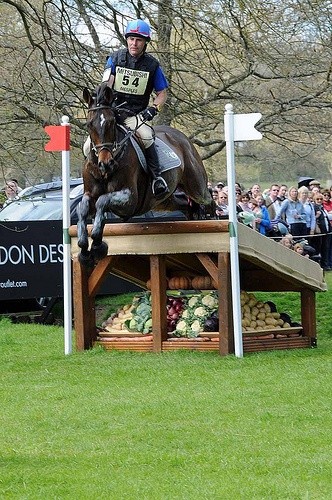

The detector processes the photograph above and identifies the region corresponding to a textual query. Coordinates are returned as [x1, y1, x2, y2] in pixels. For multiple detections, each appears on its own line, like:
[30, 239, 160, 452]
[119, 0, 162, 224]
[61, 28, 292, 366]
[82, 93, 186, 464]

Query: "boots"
[143, 143, 159, 172]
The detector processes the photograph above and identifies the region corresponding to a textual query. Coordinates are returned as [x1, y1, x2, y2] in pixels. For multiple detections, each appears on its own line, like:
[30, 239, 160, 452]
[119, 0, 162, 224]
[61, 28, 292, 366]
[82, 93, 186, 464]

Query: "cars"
[1, 177, 189, 223]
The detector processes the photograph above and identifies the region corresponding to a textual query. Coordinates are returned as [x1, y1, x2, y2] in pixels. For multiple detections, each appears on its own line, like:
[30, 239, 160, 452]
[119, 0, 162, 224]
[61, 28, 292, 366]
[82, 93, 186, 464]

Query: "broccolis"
[174, 293, 218, 333]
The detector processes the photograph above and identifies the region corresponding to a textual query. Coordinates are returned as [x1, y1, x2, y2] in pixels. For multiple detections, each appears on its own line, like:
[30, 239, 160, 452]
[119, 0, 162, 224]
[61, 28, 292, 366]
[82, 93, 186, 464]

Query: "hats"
[309, 180, 321, 186]
[217, 182, 224, 187]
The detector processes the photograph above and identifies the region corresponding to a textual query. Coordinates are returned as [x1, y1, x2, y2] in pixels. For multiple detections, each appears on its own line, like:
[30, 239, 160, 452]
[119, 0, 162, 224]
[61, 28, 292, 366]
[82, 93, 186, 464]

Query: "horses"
[76, 85, 223, 264]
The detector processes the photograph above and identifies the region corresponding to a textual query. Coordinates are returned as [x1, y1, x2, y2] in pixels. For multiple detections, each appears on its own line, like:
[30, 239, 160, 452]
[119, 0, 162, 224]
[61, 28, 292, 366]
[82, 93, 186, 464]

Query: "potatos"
[240, 291, 291, 331]
[105, 297, 137, 331]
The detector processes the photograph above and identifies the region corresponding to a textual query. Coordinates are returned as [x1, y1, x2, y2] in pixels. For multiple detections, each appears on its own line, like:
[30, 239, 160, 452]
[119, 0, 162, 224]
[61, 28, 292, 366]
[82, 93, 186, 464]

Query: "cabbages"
[125, 292, 152, 334]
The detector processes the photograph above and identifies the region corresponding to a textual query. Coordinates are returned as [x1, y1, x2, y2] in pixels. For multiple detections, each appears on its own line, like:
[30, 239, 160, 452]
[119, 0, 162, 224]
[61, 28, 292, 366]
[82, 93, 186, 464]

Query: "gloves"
[139, 106, 157, 121]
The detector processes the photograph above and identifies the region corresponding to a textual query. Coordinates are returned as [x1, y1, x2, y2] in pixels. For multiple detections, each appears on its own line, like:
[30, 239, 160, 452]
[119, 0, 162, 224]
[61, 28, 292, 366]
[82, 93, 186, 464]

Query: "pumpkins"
[191, 274, 213, 290]
[145, 275, 169, 289]
[168, 274, 192, 290]
[210, 278, 218, 290]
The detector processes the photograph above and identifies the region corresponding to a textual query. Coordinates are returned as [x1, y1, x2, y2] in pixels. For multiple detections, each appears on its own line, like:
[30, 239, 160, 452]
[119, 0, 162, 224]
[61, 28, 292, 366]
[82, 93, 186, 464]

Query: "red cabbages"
[211, 310, 219, 319]
[203, 317, 219, 331]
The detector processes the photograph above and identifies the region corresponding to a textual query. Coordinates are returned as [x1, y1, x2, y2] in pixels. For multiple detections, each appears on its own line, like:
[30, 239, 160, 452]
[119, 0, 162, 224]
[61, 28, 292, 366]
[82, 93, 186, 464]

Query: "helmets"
[125, 19, 151, 43]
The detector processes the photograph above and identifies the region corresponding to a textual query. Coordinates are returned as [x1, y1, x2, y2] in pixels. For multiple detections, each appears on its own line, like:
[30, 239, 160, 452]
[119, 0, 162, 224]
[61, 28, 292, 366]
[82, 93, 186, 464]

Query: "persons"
[83, 19, 169, 201]
[207, 180, 332, 274]
[3, 178, 23, 209]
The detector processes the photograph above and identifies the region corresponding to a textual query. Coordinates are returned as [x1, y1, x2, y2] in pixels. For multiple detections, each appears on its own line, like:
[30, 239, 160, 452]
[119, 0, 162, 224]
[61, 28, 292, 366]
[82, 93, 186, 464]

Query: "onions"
[166, 296, 187, 331]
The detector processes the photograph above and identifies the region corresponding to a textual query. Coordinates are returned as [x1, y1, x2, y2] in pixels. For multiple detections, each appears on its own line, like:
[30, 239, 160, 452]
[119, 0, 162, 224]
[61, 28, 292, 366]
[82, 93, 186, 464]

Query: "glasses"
[316, 197, 322, 201]
[242, 196, 249, 198]
[235, 193, 241, 196]
[323, 193, 330, 197]
[273, 189, 279, 192]
[220, 196, 227, 199]
